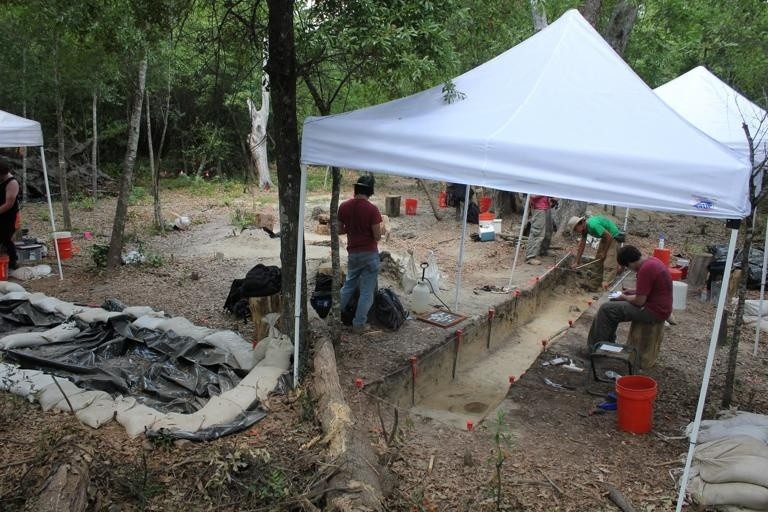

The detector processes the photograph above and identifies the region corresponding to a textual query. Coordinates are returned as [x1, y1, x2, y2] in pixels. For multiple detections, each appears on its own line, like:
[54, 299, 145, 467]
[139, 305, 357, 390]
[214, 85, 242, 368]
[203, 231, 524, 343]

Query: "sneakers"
[352, 323, 370, 332]
[542, 250, 555, 256]
[527, 258, 541, 265]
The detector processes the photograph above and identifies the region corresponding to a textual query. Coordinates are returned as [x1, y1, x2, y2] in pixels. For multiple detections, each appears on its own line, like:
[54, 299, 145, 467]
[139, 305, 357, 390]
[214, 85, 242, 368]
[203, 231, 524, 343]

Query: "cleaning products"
[479, 225, 495, 241]
[659, 234, 665, 249]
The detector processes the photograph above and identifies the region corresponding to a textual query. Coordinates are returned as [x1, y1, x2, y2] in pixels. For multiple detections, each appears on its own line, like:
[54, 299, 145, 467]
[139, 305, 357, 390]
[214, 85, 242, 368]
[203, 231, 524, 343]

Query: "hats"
[353, 176, 374, 189]
[568, 216, 585, 234]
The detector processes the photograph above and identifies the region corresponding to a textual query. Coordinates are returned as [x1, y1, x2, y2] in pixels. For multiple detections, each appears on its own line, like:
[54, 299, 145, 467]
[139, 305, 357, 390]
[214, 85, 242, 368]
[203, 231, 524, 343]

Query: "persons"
[336, 173, 386, 333]
[575, 244, 673, 361]
[0, 158, 20, 270]
[566, 215, 626, 289]
[523, 193, 561, 266]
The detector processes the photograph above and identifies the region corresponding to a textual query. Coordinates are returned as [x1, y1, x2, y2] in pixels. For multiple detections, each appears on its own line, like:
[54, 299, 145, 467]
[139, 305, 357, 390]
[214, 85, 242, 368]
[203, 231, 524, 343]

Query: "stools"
[590, 341, 638, 383]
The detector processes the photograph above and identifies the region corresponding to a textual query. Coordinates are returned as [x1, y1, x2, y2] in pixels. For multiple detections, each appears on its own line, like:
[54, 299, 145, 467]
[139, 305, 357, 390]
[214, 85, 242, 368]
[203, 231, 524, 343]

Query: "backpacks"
[223, 278, 248, 313]
[374, 287, 408, 329]
[467, 202, 480, 224]
[309, 271, 332, 317]
[243, 264, 281, 297]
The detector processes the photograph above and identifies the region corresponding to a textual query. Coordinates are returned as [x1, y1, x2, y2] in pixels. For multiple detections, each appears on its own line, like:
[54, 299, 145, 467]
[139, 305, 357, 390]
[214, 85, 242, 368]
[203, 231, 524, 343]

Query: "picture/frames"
[416, 309, 466, 329]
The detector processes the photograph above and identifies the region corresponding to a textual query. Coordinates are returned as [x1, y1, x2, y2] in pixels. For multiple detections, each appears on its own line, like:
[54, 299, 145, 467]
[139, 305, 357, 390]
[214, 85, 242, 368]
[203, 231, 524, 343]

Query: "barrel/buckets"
[479, 213, 494, 225]
[480, 196, 492, 212]
[672, 281, 688, 309]
[0, 256, 9, 281]
[412, 280, 430, 314]
[438, 192, 447, 208]
[51, 231, 72, 259]
[614, 374, 658, 433]
[668, 269, 682, 280]
[653, 247, 670, 268]
[405, 198, 417, 215]
